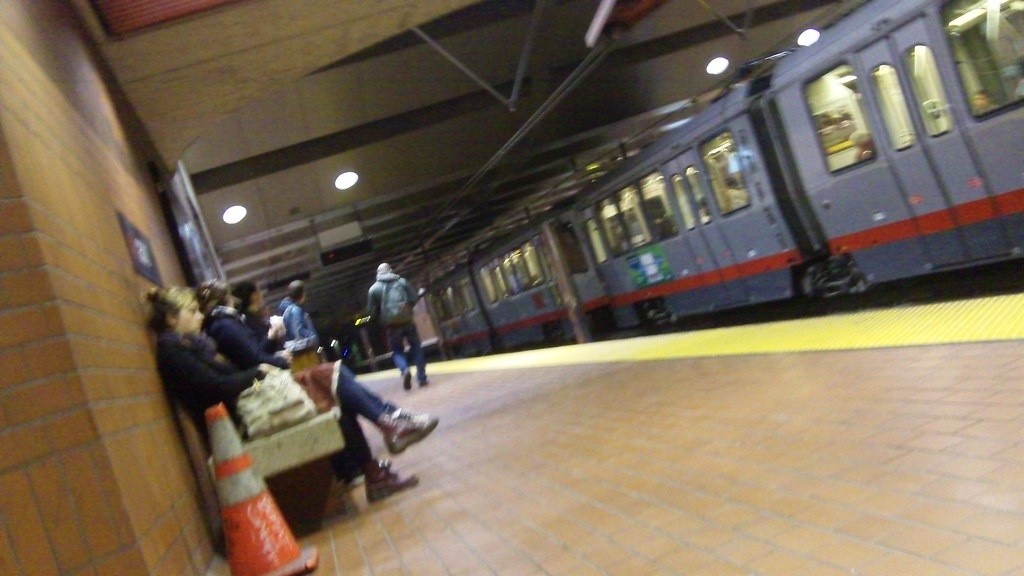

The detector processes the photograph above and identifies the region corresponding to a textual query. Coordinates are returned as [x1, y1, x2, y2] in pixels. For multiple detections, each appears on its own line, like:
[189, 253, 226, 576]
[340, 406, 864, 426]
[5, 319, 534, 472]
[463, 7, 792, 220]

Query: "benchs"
[207, 411, 346, 537]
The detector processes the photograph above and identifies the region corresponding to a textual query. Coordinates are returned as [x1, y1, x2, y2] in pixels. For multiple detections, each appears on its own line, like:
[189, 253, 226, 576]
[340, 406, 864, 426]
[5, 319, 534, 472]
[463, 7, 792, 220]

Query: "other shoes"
[403, 367, 412, 390]
[420, 381, 428, 387]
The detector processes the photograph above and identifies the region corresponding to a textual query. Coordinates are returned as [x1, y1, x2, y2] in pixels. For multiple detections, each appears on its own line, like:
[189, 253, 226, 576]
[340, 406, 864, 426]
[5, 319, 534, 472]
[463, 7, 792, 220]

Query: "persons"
[280, 280, 316, 341]
[142, 286, 439, 502]
[229, 279, 287, 354]
[197, 280, 420, 421]
[366, 263, 428, 391]
[970, 65, 1024, 112]
[847, 130, 874, 163]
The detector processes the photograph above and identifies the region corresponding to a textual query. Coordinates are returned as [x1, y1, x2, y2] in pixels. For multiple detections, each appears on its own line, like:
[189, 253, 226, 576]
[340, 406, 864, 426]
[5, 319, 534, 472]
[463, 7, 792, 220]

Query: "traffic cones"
[203, 401, 319, 576]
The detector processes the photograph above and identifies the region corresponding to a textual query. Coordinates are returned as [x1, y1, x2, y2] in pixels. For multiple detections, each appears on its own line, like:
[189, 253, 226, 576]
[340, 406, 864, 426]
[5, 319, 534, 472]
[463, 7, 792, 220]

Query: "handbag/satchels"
[235, 369, 317, 439]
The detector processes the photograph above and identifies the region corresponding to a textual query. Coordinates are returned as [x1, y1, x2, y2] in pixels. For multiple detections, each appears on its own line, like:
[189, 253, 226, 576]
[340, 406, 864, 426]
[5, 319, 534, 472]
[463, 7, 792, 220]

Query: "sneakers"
[385, 408, 440, 455]
[363, 459, 419, 502]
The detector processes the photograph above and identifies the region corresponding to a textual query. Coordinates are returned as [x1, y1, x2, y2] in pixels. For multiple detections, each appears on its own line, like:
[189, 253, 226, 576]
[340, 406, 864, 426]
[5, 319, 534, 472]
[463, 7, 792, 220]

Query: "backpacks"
[381, 278, 412, 326]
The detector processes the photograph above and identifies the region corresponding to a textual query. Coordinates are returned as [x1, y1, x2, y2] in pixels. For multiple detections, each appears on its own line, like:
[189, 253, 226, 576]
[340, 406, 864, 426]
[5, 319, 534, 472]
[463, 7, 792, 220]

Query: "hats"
[197, 280, 229, 317]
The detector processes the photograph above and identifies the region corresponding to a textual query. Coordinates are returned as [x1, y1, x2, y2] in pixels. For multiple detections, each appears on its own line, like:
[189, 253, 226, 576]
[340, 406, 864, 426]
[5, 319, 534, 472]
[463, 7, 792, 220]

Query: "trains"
[420, 1, 1024, 358]
